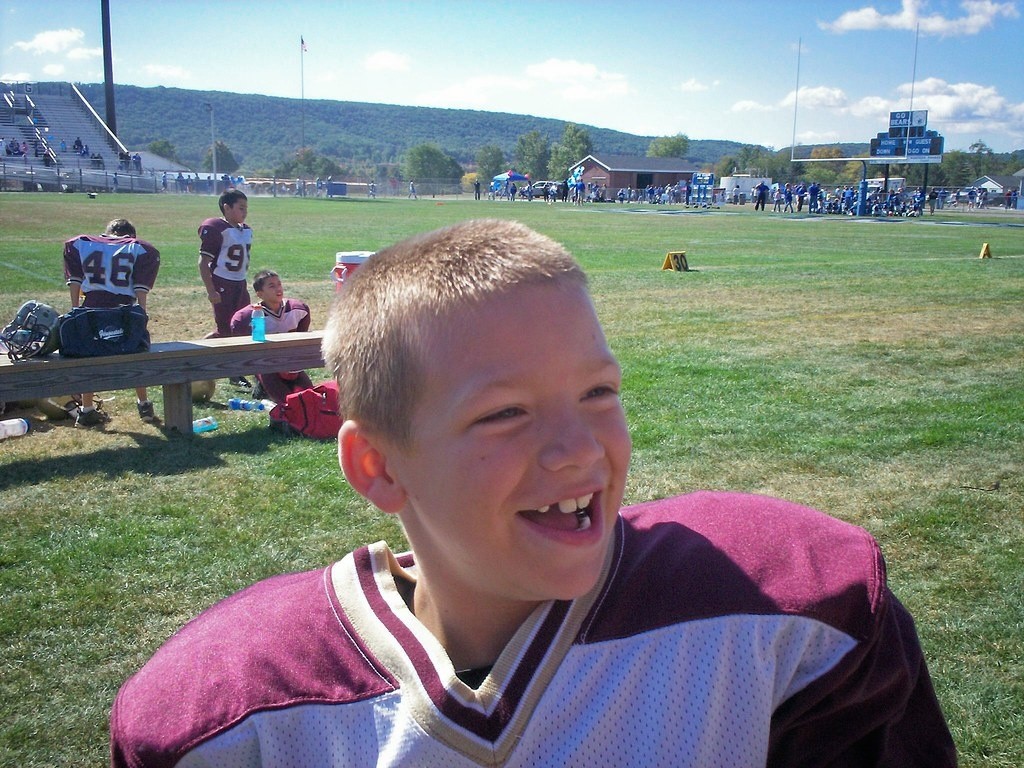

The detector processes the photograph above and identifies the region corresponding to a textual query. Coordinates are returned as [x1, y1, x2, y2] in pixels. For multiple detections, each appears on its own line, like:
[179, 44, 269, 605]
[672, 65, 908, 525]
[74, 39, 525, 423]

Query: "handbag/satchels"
[58, 305, 152, 357]
[269, 383, 339, 439]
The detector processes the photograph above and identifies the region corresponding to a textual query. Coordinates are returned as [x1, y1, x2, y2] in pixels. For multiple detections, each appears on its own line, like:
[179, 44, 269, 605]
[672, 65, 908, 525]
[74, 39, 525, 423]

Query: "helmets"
[0, 300, 60, 363]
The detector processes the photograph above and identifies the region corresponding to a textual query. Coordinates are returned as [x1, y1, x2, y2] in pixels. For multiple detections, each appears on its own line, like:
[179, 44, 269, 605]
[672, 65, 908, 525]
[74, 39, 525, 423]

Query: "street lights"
[202, 101, 218, 196]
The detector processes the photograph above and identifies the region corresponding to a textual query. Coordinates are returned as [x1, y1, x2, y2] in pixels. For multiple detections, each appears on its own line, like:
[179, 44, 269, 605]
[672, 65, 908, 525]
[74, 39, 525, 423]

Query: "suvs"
[524, 180, 556, 198]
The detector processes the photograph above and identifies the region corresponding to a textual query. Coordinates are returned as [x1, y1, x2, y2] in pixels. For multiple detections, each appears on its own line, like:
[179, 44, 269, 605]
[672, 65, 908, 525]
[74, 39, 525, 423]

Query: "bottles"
[228, 398, 264, 411]
[192, 415, 219, 433]
[0, 418, 31, 440]
[251, 302, 267, 342]
[5, 330, 43, 343]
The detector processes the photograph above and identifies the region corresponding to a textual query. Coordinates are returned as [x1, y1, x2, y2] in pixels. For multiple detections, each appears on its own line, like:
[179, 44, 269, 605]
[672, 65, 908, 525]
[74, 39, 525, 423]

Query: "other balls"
[191, 381, 217, 403]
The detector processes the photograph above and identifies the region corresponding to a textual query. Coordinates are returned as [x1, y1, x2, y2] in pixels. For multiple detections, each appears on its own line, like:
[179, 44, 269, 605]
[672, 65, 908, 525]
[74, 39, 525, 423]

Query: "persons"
[118, 151, 142, 172]
[527, 180, 533, 201]
[509, 183, 517, 201]
[0, 137, 29, 157]
[474, 179, 480, 200]
[295, 174, 334, 198]
[1005, 189, 1017, 209]
[733, 185, 740, 205]
[113, 172, 118, 193]
[229, 271, 313, 402]
[409, 179, 417, 198]
[197, 187, 251, 388]
[63, 220, 154, 429]
[618, 182, 681, 205]
[544, 179, 607, 206]
[43, 150, 50, 168]
[370, 181, 376, 199]
[177, 172, 243, 194]
[751, 181, 989, 217]
[61, 137, 104, 169]
[112, 219, 956, 768]
[161, 171, 167, 193]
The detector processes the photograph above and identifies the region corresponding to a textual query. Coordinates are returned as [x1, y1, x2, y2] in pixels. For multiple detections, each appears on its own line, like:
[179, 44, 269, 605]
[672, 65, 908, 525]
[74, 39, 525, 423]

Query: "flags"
[302, 39, 307, 51]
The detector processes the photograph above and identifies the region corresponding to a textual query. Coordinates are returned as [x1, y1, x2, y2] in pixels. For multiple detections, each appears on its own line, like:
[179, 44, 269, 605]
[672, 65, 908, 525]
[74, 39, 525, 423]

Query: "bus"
[862, 177, 906, 193]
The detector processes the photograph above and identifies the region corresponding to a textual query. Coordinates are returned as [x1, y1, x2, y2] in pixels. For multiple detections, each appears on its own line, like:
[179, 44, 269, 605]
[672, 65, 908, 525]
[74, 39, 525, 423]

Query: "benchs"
[0, 91, 126, 170]
[0, 331, 324, 435]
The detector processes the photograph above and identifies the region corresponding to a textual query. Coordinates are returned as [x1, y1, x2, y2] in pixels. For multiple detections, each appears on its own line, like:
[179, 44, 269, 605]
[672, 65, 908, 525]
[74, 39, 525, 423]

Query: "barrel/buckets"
[331, 251, 376, 293]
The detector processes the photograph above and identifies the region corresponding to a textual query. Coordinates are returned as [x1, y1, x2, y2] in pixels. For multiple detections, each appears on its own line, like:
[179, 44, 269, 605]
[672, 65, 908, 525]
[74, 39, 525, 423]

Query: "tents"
[494, 171, 529, 199]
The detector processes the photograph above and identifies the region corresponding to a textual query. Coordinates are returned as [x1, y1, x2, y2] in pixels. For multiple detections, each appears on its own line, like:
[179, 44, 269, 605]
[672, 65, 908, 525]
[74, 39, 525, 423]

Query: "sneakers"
[230, 374, 252, 388]
[136, 401, 154, 422]
[75, 410, 107, 430]
